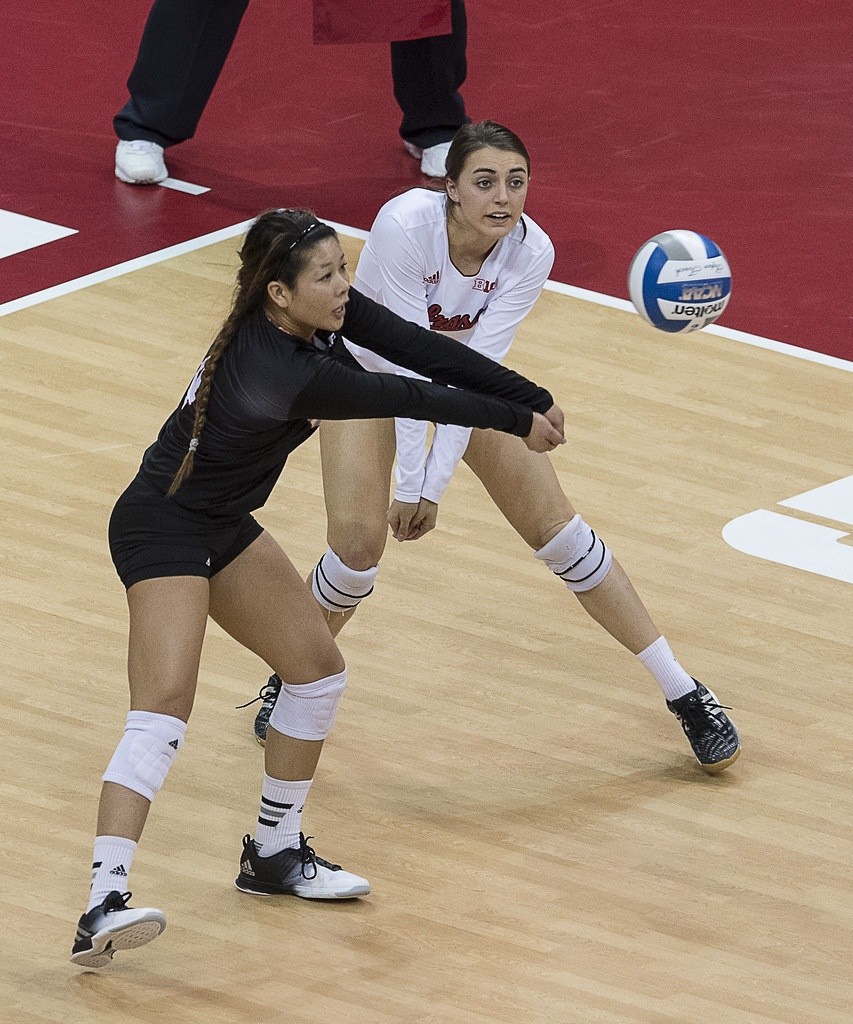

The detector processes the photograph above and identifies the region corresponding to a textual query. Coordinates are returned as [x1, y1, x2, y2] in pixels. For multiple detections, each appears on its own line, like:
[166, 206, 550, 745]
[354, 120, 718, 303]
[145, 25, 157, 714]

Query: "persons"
[68, 209, 568, 967]
[235, 122, 742, 771]
[113, 0, 470, 185]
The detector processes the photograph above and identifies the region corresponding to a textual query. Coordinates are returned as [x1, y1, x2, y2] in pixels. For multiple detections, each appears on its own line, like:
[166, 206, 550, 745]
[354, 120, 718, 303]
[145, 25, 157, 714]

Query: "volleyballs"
[628, 229, 733, 333]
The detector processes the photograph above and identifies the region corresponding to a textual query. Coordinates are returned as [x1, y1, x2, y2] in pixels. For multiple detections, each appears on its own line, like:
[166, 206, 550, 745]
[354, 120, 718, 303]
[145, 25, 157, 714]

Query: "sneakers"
[114, 138, 167, 184]
[69, 889, 169, 969]
[666, 673, 744, 774]
[234, 830, 371, 899]
[235, 674, 283, 748]
[401, 137, 453, 181]
